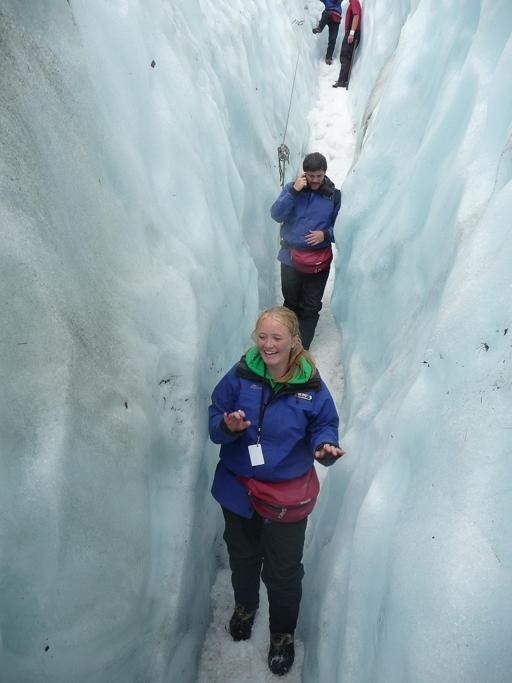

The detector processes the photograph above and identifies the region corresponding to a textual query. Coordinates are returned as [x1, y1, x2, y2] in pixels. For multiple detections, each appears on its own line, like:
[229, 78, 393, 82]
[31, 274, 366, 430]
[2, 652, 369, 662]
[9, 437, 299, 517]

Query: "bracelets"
[349, 29, 355, 35]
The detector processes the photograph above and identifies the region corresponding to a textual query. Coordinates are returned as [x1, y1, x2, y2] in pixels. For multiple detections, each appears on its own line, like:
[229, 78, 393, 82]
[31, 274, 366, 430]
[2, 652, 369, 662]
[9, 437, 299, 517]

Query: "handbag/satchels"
[281, 242, 334, 274]
[331, 10, 341, 23]
[216, 465, 319, 525]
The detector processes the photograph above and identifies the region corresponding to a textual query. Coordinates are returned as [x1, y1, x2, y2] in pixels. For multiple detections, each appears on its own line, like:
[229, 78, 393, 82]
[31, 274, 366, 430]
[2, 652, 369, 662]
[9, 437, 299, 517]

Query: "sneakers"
[333, 80, 348, 88]
[313, 27, 320, 33]
[325, 56, 332, 63]
[267, 631, 295, 675]
[228, 606, 257, 639]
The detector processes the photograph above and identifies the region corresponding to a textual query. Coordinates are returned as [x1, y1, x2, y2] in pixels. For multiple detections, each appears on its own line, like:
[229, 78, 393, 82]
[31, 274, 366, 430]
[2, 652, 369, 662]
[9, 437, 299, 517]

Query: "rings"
[237, 408, 241, 411]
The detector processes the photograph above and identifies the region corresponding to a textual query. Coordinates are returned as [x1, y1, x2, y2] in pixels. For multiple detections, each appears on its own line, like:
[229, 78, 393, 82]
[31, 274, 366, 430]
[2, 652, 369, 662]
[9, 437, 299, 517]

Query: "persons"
[313, 0, 343, 65]
[208, 306, 349, 678]
[269, 151, 342, 353]
[332, 0, 363, 89]
